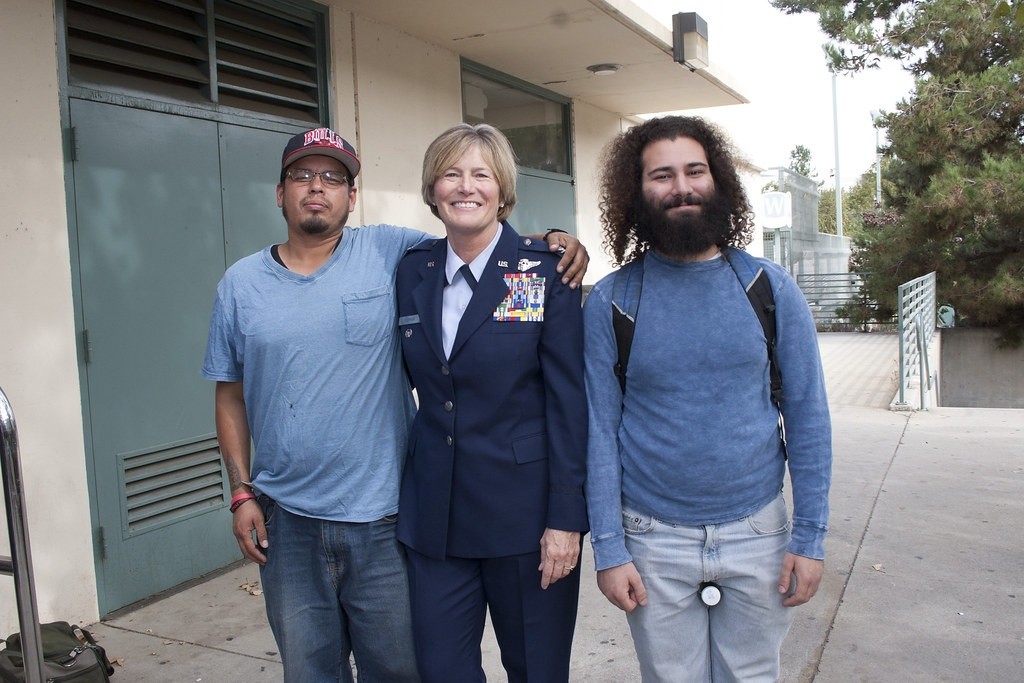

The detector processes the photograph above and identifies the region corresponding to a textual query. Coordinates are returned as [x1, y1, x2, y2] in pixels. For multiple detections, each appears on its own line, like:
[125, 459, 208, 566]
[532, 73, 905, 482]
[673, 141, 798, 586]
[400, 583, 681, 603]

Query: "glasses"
[285, 168, 351, 188]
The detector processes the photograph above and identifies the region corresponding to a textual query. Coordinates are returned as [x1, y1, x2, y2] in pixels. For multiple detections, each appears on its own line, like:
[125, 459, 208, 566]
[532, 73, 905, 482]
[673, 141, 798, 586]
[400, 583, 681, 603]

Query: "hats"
[282, 127, 361, 178]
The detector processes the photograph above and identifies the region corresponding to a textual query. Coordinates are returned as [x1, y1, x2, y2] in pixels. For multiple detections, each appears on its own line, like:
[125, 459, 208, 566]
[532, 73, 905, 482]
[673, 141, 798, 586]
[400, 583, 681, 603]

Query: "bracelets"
[229, 494, 256, 512]
[543, 228, 568, 241]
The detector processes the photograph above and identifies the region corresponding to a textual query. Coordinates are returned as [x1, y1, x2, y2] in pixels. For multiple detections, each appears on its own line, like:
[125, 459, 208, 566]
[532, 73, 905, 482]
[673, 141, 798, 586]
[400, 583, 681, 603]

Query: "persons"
[201, 127, 591, 683]
[393, 126, 591, 683]
[583, 116, 830, 683]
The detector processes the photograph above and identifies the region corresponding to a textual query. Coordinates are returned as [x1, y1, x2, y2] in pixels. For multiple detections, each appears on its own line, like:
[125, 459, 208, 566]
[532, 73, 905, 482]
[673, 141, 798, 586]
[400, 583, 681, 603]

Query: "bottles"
[698, 582, 722, 606]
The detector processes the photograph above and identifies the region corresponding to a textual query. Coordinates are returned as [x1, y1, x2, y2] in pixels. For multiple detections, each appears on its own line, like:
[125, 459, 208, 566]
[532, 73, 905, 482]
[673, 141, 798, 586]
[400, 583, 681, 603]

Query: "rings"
[564, 567, 573, 571]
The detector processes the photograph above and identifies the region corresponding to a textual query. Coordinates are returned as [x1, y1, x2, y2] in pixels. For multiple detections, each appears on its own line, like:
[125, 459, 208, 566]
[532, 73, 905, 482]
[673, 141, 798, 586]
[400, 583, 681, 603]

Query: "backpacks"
[0, 621, 114, 683]
